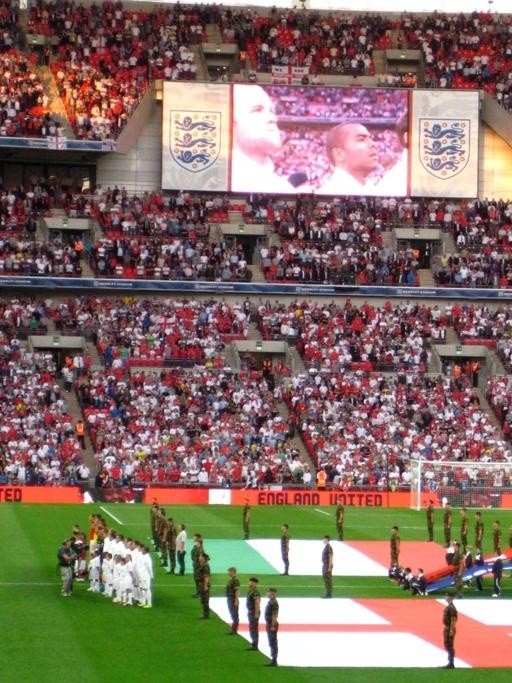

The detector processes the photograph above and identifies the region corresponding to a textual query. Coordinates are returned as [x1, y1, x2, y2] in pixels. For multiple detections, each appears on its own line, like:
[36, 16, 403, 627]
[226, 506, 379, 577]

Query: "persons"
[387, 499, 512, 600]
[1, 1, 512, 509]
[280, 522, 289, 576]
[334, 496, 346, 540]
[152, 502, 211, 621]
[264, 586, 279, 667]
[242, 498, 253, 539]
[321, 535, 333, 599]
[225, 566, 239, 635]
[56, 513, 153, 610]
[443, 592, 459, 668]
[245, 577, 261, 651]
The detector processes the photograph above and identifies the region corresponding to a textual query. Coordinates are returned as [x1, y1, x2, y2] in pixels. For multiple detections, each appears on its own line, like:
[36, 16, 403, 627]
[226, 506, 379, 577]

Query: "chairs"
[0, 0, 512, 501]
